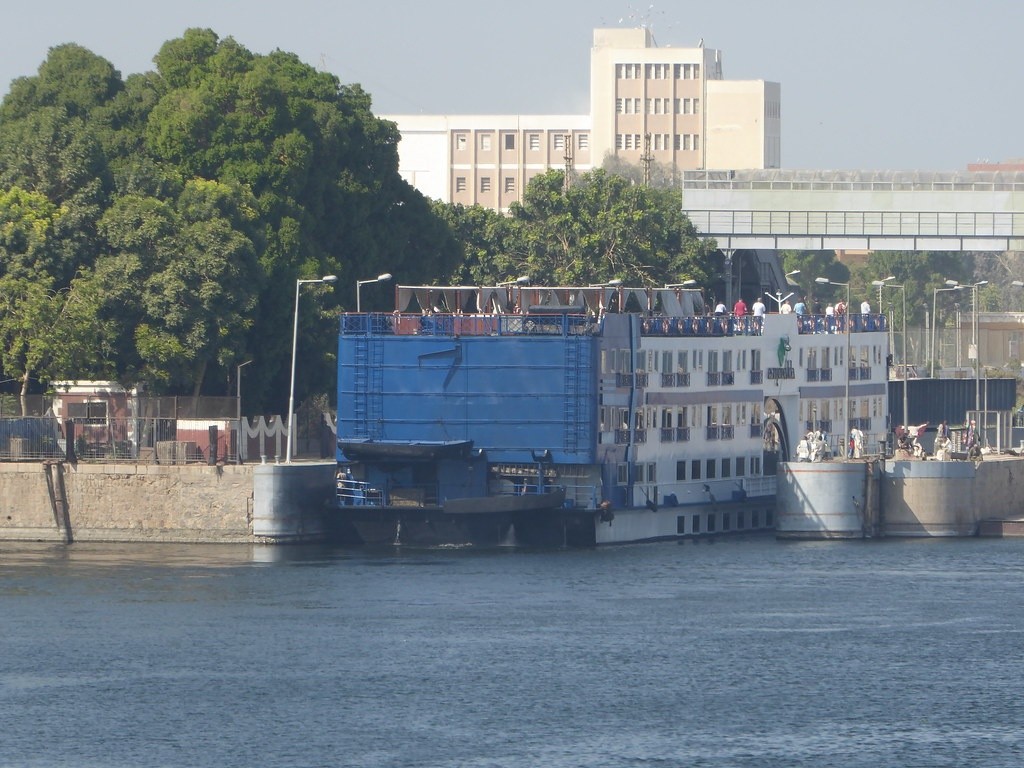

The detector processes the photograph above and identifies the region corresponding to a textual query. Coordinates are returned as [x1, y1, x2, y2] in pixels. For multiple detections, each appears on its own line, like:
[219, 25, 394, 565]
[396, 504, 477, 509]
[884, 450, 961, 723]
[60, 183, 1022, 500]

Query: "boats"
[335, 310, 893, 551]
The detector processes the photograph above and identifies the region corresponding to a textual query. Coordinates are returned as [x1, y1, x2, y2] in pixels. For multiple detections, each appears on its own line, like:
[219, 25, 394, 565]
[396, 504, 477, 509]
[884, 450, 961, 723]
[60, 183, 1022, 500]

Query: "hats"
[757, 297, 762, 301]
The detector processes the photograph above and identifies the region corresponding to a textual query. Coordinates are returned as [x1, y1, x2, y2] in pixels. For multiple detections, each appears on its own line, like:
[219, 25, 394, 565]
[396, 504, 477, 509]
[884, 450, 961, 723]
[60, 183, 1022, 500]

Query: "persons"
[812, 298, 825, 334]
[825, 302, 835, 334]
[861, 299, 871, 327]
[835, 299, 848, 333]
[715, 301, 727, 316]
[752, 298, 766, 316]
[793, 298, 806, 315]
[734, 298, 747, 317]
[794, 419, 983, 469]
[781, 300, 792, 314]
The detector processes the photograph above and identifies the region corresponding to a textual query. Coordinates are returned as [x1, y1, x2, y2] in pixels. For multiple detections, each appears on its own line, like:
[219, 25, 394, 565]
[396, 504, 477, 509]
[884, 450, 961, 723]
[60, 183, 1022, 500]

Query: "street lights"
[496, 277, 531, 288]
[666, 279, 697, 288]
[972, 281, 989, 375]
[284, 277, 338, 463]
[588, 280, 622, 289]
[358, 272, 391, 310]
[236, 361, 252, 465]
[945, 280, 979, 436]
[872, 280, 908, 437]
[815, 277, 850, 459]
[879, 275, 896, 314]
[930, 286, 964, 378]
